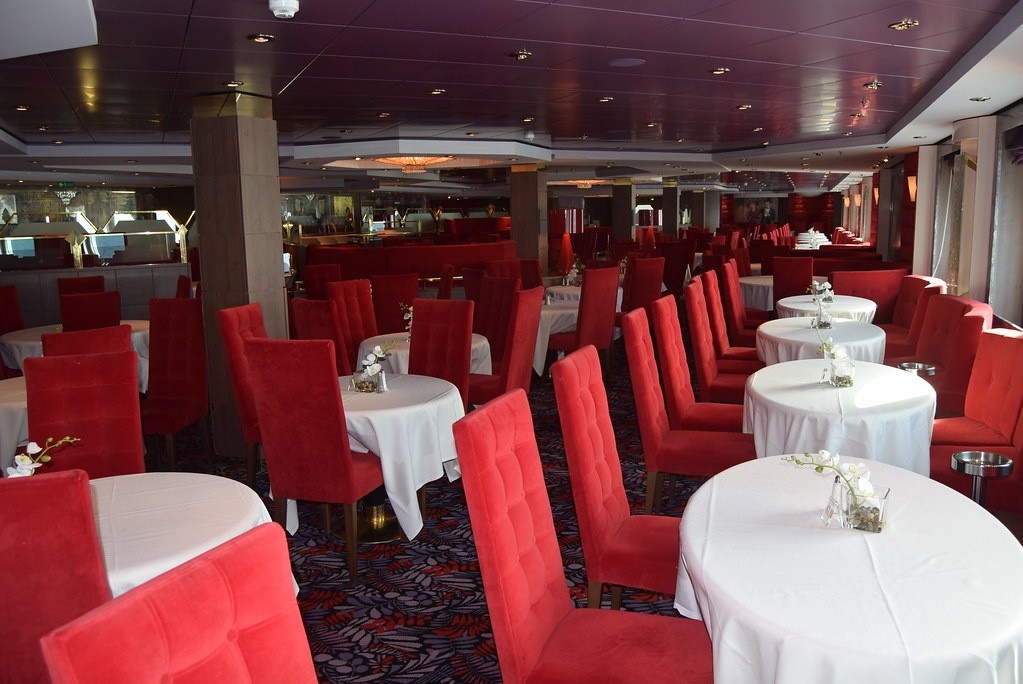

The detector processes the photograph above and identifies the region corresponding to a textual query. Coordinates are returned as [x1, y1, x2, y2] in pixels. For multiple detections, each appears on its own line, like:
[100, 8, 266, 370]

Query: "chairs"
[0, 222, 1023, 684]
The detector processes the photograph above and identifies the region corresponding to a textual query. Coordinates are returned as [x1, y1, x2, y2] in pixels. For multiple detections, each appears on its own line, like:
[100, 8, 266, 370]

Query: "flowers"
[780, 450, 875, 518]
[361, 338, 399, 377]
[805, 281, 823, 319]
[820, 282, 832, 292]
[816, 327, 837, 360]
[399, 302, 413, 335]
[7, 436, 81, 478]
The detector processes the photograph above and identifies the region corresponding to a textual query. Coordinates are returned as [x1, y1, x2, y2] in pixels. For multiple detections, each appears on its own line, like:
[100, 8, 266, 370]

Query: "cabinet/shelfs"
[0, 262, 195, 329]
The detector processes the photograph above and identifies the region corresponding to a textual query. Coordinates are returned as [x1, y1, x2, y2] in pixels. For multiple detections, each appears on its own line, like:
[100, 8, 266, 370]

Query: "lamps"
[369, 156, 456, 173]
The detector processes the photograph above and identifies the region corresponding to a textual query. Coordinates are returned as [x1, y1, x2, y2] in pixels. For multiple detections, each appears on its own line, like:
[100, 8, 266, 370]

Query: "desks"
[268, 374, 465, 545]
[533, 299, 580, 378]
[0, 376, 28, 478]
[89, 472, 299, 598]
[776, 295, 877, 323]
[674, 453, 1023, 684]
[739, 275, 828, 319]
[356, 332, 492, 375]
[545, 285, 623, 340]
[0, 320, 149, 393]
[756, 316, 886, 365]
[742, 359, 936, 477]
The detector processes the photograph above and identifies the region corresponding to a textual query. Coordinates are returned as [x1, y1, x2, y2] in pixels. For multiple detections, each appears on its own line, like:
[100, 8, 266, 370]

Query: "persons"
[746, 197, 776, 224]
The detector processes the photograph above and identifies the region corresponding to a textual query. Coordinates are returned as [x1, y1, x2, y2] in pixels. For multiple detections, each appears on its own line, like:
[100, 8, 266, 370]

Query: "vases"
[829, 359, 855, 387]
[846, 486, 890, 533]
[816, 313, 832, 329]
[822, 292, 834, 303]
[352, 371, 379, 393]
